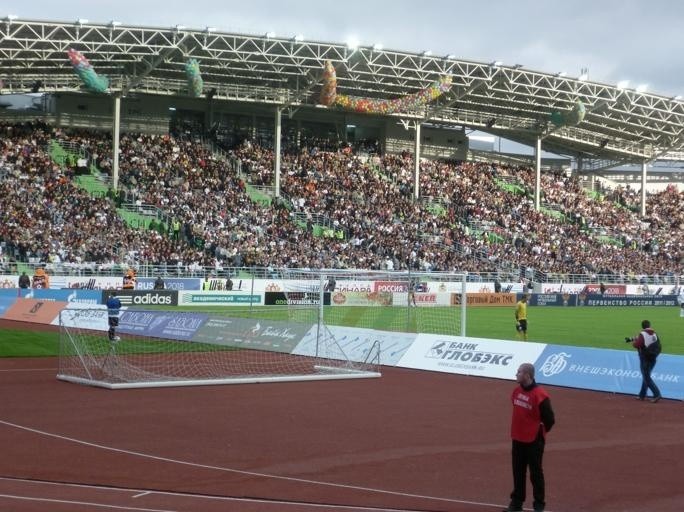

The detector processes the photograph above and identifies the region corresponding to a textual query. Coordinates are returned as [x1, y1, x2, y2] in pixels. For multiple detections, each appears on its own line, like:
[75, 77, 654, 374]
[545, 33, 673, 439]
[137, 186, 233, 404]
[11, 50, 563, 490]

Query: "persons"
[632, 319, 663, 404]
[502, 361, 556, 512]
[514, 293, 530, 342]
[106, 292, 122, 341]
[1, 117, 684, 297]
[677, 291, 684, 318]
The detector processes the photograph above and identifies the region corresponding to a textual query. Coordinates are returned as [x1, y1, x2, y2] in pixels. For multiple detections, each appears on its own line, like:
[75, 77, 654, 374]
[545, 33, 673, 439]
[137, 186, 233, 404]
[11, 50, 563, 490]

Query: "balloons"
[185, 58, 203, 99]
[66, 46, 109, 93]
[319, 60, 454, 116]
